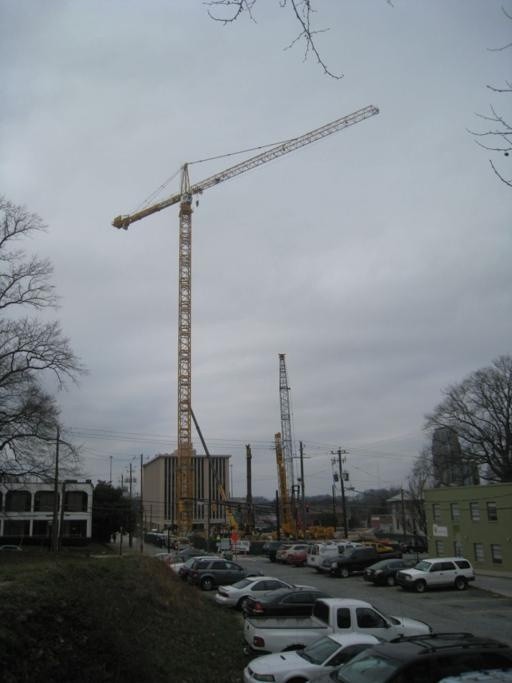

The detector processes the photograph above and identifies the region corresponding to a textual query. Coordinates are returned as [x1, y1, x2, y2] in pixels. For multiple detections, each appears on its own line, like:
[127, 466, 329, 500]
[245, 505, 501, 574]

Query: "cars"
[307, 633, 512, 683]
[242, 632, 387, 683]
[0, 545, 23, 551]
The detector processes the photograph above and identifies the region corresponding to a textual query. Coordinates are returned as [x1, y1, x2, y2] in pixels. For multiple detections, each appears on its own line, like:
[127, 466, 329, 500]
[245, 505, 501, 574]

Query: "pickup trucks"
[245, 598, 431, 656]
